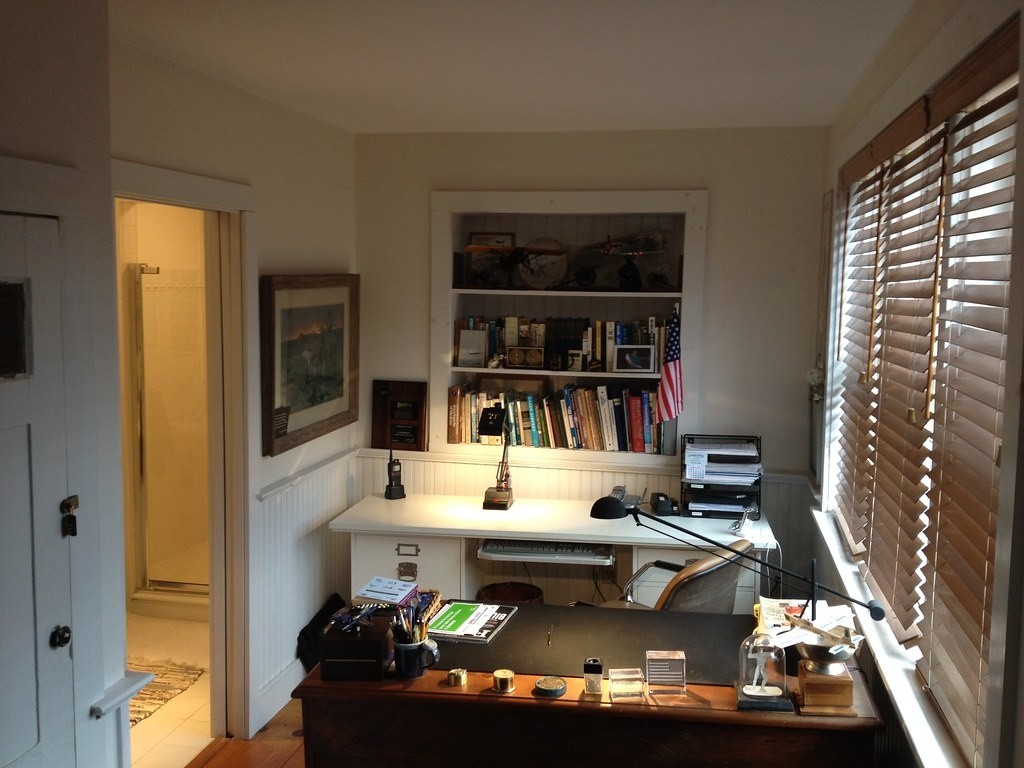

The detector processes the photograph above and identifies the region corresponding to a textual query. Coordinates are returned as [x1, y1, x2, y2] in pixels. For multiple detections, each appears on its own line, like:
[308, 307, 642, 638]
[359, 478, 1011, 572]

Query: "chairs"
[567, 540, 755, 614]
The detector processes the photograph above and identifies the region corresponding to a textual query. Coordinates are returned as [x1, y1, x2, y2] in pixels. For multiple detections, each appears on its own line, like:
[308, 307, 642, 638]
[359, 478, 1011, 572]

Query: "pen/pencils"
[389, 606, 428, 644]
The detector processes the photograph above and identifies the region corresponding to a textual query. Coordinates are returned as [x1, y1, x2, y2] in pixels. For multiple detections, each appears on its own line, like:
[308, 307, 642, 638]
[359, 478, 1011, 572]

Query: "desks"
[330, 493, 777, 615]
[291, 601, 883, 768]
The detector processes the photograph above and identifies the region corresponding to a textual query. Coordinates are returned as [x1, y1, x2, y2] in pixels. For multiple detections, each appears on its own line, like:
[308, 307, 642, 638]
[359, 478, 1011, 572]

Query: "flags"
[653, 304, 684, 428]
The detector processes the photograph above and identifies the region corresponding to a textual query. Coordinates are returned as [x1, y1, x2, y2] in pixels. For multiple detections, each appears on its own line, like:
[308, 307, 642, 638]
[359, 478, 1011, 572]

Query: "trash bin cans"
[476, 582, 544, 604]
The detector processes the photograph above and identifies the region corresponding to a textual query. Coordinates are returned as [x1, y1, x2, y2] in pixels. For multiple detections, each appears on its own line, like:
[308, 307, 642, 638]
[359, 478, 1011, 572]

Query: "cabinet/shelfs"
[428, 188, 710, 465]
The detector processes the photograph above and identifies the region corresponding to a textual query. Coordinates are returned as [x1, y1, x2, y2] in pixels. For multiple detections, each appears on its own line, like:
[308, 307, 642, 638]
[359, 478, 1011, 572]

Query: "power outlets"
[601, 559, 617, 584]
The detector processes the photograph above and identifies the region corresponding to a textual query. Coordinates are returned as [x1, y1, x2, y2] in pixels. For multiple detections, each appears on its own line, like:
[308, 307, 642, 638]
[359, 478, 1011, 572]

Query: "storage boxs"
[798, 660, 854, 707]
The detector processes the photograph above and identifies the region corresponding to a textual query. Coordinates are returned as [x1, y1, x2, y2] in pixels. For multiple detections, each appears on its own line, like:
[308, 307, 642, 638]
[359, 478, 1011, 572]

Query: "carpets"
[125, 653, 208, 729]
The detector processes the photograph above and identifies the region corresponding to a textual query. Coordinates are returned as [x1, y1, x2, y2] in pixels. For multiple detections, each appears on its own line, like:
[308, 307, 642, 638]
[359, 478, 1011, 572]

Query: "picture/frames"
[613, 345, 654, 372]
[263, 273, 360, 457]
[506, 346, 545, 368]
[469, 232, 516, 290]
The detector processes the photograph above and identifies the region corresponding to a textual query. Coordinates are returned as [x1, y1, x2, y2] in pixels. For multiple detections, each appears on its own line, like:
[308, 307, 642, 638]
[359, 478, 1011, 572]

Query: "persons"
[748, 644, 780, 687]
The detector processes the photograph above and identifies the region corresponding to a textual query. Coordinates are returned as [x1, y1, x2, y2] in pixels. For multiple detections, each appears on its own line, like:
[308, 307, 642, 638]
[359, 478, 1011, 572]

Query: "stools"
[478, 582, 544, 605]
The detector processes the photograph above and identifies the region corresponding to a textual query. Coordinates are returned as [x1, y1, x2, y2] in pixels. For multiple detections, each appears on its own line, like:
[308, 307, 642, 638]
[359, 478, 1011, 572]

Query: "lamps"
[590, 496, 885, 675]
[478, 402, 514, 510]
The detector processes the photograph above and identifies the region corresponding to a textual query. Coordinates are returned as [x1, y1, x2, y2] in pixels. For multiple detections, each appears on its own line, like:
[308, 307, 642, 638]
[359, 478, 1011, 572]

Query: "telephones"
[609, 485, 648, 509]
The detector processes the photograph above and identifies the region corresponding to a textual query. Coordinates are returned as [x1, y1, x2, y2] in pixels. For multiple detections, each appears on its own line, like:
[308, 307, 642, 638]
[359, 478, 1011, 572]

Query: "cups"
[392, 634, 440, 681]
[551, 350, 574, 371]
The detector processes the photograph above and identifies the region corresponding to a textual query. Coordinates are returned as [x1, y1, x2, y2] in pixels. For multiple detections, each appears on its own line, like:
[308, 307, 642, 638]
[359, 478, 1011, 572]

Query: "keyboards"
[482, 540, 597, 559]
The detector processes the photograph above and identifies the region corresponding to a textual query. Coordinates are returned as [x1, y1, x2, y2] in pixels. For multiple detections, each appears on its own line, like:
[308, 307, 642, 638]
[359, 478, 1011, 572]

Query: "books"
[454, 316, 667, 373]
[448, 381, 676, 455]
[426, 601, 518, 644]
